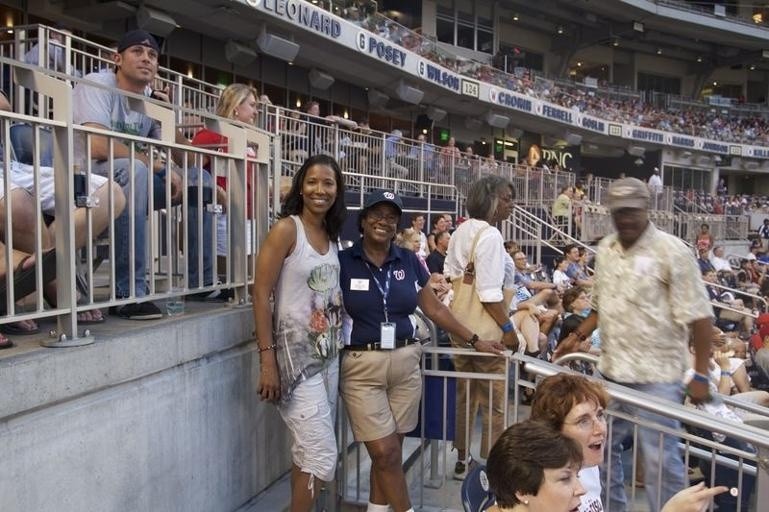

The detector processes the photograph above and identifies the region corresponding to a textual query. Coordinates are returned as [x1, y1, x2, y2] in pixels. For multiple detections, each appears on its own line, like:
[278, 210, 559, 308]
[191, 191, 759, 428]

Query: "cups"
[165, 288, 185, 318]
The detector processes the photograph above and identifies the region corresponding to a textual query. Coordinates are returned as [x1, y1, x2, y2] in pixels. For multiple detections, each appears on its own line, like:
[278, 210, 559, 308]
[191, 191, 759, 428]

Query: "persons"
[1, 1, 769, 511]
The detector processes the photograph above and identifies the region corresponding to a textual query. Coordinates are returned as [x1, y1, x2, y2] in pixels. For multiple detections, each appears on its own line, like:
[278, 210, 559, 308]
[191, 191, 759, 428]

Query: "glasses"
[576, 408, 609, 429]
[518, 257, 528, 260]
[368, 211, 398, 224]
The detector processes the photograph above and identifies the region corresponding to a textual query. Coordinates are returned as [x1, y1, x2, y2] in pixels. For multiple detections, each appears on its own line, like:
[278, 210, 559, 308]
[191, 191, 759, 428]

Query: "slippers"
[45, 288, 106, 323]
[0, 247, 56, 311]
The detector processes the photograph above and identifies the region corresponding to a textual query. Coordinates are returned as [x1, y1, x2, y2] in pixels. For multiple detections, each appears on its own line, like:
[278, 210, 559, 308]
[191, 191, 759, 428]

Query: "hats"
[605, 177, 651, 214]
[363, 188, 404, 215]
[114, 30, 160, 54]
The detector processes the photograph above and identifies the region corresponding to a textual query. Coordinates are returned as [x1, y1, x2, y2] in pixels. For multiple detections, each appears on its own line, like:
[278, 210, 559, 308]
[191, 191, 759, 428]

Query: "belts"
[340, 335, 417, 353]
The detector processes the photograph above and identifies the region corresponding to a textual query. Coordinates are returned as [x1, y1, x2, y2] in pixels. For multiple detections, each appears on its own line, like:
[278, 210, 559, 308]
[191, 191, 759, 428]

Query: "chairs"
[8, 120, 112, 299]
[460, 464, 495, 512]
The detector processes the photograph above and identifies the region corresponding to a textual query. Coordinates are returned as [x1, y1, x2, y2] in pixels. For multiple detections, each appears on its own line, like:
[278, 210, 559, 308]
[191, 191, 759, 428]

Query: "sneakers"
[453, 459, 489, 481]
[182, 283, 236, 302]
[0, 318, 41, 335]
[107, 296, 162, 320]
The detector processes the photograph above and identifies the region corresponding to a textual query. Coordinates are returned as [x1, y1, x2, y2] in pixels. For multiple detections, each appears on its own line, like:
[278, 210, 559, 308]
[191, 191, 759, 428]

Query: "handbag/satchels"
[445, 275, 519, 351]
